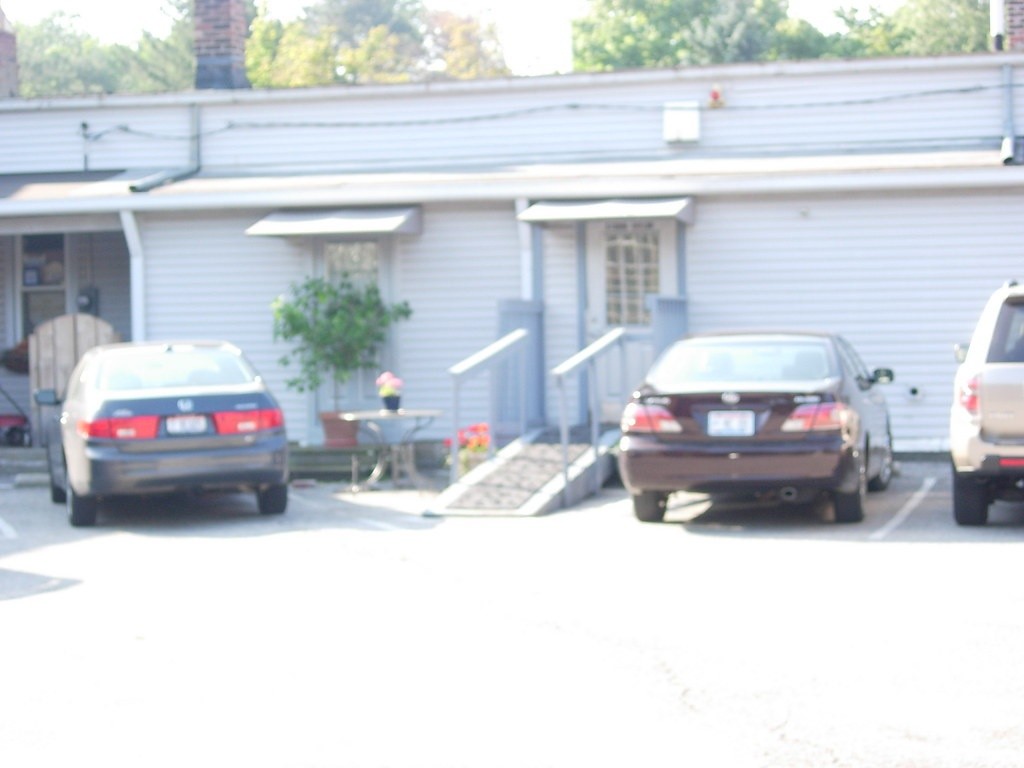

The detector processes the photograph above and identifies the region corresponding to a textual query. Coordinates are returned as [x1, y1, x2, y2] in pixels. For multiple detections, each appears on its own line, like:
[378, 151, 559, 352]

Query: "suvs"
[947, 281, 1023, 529]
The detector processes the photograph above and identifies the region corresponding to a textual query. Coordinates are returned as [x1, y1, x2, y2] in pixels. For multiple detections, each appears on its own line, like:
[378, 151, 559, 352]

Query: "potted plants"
[273, 269, 418, 448]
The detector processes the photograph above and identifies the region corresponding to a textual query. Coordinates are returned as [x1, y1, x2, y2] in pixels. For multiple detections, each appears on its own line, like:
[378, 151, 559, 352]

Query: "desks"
[340, 409, 440, 494]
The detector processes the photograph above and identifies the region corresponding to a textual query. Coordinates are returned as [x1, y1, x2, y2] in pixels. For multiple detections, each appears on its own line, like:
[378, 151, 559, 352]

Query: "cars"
[619, 328, 897, 524]
[32, 338, 291, 527]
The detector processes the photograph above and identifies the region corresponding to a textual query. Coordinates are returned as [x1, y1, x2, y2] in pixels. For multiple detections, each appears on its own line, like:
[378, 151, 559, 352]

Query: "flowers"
[375, 372, 404, 397]
[442, 423, 492, 477]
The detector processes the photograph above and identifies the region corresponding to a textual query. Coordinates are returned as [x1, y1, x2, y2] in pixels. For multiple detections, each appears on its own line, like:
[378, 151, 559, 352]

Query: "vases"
[385, 396, 401, 411]
